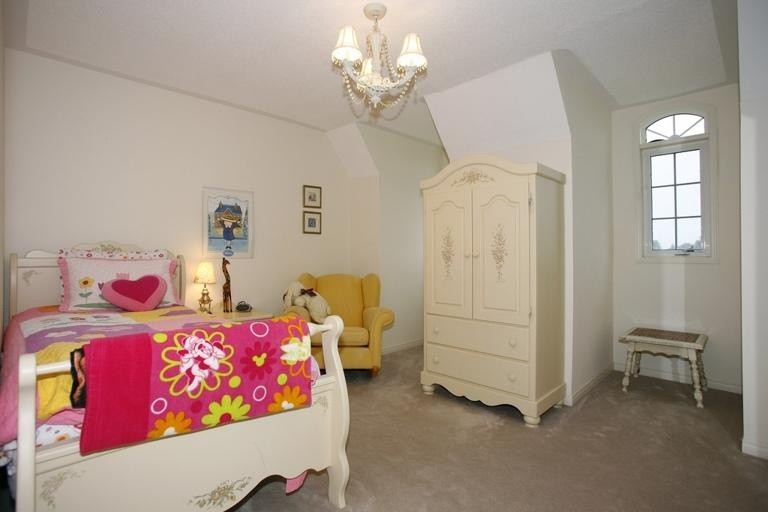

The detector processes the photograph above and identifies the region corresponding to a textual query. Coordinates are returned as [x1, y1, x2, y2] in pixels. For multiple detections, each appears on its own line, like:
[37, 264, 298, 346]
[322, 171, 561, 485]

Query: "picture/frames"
[302, 183, 322, 235]
[201, 183, 255, 260]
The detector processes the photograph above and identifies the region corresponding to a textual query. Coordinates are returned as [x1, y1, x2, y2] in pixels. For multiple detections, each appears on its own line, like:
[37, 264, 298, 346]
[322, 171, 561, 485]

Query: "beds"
[0, 238, 353, 512]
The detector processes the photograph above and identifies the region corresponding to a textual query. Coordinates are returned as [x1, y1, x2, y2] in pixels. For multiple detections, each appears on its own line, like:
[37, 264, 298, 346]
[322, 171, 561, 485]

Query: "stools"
[618, 326, 710, 410]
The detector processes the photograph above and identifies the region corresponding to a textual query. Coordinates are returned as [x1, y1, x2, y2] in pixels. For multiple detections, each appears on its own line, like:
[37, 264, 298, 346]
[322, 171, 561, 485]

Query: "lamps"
[328, 1, 429, 110]
[189, 260, 219, 315]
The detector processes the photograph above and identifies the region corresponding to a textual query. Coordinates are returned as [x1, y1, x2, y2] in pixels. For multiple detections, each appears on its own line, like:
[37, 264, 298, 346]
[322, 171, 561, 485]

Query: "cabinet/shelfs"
[415, 152, 568, 428]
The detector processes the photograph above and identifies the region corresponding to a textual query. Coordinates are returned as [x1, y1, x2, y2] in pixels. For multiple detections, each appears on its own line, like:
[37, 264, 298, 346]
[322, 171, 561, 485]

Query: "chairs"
[286, 268, 397, 381]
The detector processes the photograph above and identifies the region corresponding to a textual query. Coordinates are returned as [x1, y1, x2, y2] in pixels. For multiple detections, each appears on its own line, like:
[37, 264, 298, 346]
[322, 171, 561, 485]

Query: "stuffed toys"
[280, 280, 332, 324]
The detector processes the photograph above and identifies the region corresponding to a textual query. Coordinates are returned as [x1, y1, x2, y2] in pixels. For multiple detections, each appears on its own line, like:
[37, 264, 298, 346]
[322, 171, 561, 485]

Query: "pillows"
[53, 256, 184, 314]
